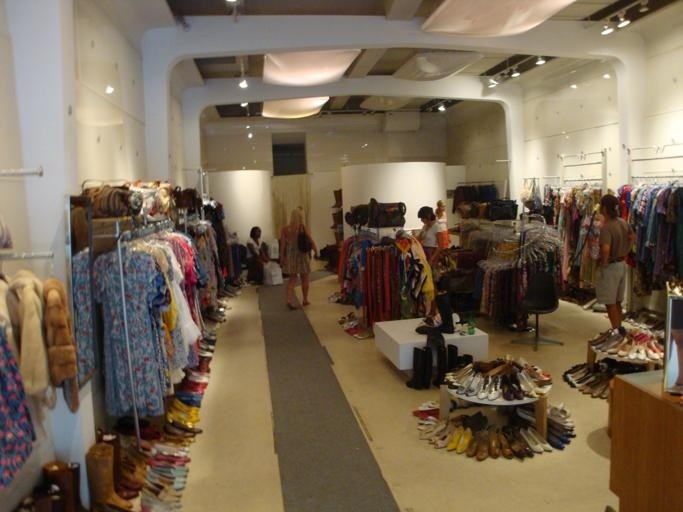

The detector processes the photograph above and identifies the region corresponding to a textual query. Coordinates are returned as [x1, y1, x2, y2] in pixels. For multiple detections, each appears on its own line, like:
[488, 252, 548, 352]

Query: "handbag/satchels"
[521, 179, 542, 211]
[82, 180, 202, 218]
[297, 232, 311, 253]
[457, 200, 518, 221]
[368, 199, 406, 228]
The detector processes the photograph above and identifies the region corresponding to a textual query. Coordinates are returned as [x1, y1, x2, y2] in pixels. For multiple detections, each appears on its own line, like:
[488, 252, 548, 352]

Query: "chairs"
[510, 274, 565, 352]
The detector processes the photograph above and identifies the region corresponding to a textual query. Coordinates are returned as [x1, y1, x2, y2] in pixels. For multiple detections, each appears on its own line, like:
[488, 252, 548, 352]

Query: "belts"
[360, 246, 400, 328]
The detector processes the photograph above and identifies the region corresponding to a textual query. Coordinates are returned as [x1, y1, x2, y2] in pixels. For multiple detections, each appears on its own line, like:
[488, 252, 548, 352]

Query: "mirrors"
[69, 195, 101, 390]
[662, 293, 682, 394]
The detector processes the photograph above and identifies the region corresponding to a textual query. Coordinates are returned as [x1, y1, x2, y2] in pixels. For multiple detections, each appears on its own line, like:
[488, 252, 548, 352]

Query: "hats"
[601, 195, 618, 217]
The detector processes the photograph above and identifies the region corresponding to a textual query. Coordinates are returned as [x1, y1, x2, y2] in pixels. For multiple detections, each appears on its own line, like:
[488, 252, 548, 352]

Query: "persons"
[433, 198, 452, 248]
[591, 191, 638, 329]
[278, 206, 320, 309]
[413, 205, 444, 265]
[243, 225, 271, 286]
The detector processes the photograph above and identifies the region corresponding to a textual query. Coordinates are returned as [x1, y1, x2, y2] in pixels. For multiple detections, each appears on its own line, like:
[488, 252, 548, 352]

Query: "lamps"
[601, 0, 650, 36]
[488, 55, 546, 88]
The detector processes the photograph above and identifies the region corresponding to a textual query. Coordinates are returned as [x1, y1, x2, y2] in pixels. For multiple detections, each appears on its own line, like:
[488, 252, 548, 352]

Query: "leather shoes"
[338, 312, 373, 339]
[443, 352, 553, 401]
[114, 332, 217, 512]
[563, 358, 659, 398]
[590, 317, 665, 361]
[212, 278, 251, 323]
[413, 398, 576, 460]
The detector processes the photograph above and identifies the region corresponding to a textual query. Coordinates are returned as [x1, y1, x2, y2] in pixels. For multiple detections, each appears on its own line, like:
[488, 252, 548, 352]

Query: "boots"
[330, 189, 344, 243]
[435, 293, 454, 333]
[416, 326, 444, 346]
[406, 344, 473, 390]
[32, 433, 142, 512]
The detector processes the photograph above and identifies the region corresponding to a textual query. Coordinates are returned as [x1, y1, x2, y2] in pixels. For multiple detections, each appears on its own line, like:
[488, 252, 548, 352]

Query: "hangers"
[477, 214, 558, 273]
[0, 252, 54, 279]
